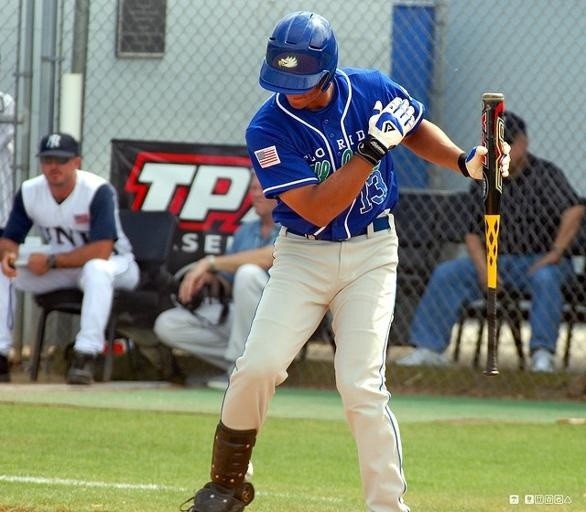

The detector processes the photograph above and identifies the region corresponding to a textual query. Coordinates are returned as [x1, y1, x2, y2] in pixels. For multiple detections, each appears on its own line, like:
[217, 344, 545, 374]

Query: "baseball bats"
[480, 92, 506, 375]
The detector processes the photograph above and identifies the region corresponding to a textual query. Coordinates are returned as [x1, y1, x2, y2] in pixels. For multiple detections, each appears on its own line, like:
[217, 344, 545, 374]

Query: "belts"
[286, 218, 388, 240]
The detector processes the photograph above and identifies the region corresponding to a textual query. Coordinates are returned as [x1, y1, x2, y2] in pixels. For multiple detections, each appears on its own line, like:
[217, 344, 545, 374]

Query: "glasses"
[42, 156, 70, 164]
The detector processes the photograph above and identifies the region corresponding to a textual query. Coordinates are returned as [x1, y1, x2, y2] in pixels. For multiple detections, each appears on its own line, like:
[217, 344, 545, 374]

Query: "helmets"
[260, 11, 338, 95]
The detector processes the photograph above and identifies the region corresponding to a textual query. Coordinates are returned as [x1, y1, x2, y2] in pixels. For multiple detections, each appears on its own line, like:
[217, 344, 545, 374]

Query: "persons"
[151, 163, 291, 394]
[0, 133, 142, 385]
[177, 10, 513, 510]
[394, 112, 584, 375]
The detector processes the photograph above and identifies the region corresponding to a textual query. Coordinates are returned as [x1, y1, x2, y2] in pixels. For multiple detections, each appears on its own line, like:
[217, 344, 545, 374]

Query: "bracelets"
[205, 253, 216, 273]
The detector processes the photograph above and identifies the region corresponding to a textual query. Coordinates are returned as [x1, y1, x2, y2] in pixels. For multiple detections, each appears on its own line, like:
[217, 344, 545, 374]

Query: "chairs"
[29, 211, 181, 385]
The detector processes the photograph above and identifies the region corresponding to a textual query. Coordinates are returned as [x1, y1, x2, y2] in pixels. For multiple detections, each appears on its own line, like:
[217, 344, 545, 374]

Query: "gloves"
[459, 143, 511, 180]
[356, 96, 415, 166]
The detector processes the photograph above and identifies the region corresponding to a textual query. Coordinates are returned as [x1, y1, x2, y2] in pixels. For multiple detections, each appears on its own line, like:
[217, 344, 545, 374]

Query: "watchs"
[46, 252, 57, 269]
[552, 244, 563, 257]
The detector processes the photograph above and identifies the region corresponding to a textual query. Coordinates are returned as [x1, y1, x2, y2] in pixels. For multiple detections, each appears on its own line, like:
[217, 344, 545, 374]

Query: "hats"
[37, 133, 79, 160]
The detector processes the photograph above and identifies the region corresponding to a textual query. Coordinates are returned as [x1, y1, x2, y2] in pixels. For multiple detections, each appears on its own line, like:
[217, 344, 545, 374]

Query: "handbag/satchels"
[96, 325, 186, 384]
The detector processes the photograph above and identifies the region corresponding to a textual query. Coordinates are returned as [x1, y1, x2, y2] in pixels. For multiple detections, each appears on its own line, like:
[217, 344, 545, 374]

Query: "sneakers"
[68, 352, 95, 383]
[0, 355, 10, 382]
[192, 482, 244, 511]
[396, 349, 445, 367]
[532, 352, 554, 372]
[209, 370, 288, 389]
